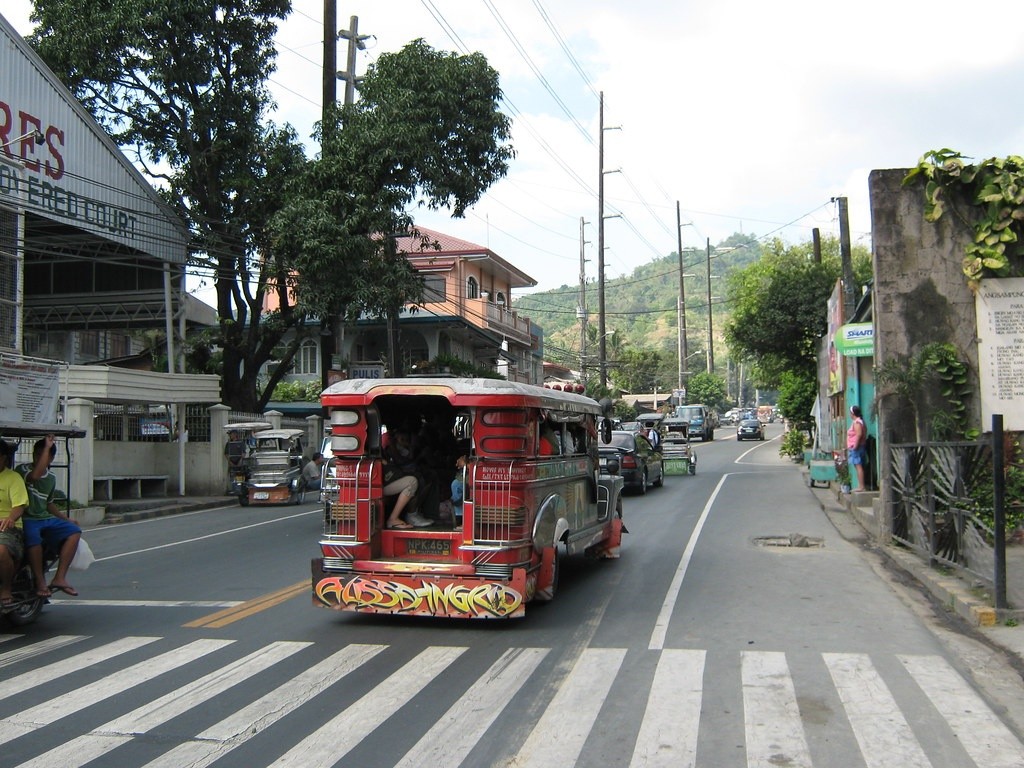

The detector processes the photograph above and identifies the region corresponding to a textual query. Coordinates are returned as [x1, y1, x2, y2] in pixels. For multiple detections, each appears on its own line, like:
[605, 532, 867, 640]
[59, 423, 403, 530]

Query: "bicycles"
[781, 419, 784, 424]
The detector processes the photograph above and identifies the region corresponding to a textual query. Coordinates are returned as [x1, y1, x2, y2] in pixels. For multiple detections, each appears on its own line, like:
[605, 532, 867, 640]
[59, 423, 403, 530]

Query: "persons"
[14, 433, 82, 596]
[539, 420, 587, 461]
[380, 407, 471, 532]
[847, 405, 866, 492]
[171, 420, 189, 443]
[642, 421, 658, 450]
[302, 453, 323, 504]
[828, 341, 841, 394]
[224, 430, 247, 495]
[0, 437, 29, 604]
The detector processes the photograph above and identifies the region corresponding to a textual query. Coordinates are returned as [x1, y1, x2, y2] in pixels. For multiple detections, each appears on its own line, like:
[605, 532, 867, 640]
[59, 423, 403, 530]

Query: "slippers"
[0, 582, 11, 605]
[386, 522, 416, 530]
[35, 582, 52, 597]
[51, 578, 78, 596]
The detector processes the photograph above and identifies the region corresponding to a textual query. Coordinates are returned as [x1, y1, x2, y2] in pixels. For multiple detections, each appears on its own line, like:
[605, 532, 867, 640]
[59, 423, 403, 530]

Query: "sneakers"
[405, 511, 436, 527]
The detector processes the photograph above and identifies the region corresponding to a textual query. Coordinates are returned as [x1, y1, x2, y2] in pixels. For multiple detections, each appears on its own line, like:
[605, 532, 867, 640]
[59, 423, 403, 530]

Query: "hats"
[381, 423, 388, 435]
[227, 430, 238, 434]
[449, 440, 471, 457]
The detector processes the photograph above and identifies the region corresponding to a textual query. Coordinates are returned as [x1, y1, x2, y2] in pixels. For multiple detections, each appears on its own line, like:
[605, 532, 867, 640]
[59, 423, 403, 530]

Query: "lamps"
[0, 128, 46, 148]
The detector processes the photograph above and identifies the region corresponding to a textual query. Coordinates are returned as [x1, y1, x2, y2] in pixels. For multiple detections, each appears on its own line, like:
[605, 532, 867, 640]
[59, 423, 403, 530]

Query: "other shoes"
[453, 526, 463, 531]
[850, 488, 866, 493]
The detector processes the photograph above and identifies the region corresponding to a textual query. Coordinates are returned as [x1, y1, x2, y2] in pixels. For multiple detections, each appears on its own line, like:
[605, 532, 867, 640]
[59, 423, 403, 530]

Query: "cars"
[736, 419, 766, 442]
[719, 415, 734, 426]
[616, 421, 643, 430]
[319, 428, 337, 523]
[595, 430, 664, 495]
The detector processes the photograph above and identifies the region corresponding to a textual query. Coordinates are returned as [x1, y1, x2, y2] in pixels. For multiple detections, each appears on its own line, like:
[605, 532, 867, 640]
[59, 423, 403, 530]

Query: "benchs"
[93, 474, 170, 500]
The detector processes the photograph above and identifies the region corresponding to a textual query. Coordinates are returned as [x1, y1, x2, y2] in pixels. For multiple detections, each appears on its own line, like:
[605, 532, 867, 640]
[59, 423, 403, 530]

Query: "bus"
[731, 407, 757, 420]
[309, 371, 625, 620]
[757, 408, 774, 424]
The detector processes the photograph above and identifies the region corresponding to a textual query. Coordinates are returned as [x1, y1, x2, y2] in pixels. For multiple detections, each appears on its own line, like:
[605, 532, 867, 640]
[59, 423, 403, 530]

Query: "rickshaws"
[0, 421, 87, 626]
[221, 422, 307, 505]
[647, 412, 697, 475]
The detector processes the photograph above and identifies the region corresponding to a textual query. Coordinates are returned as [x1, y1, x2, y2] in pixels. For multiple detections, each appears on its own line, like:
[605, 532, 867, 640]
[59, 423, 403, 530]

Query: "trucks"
[674, 405, 720, 442]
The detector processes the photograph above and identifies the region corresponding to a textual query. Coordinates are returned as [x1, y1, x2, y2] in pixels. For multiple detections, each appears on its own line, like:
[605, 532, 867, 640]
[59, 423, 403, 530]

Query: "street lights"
[579, 331, 615, 395]
[678, 351, 701, 404]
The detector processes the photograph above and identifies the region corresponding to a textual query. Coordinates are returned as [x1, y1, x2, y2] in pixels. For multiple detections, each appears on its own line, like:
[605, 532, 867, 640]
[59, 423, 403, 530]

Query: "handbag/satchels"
[69, 537, 96, 571]
